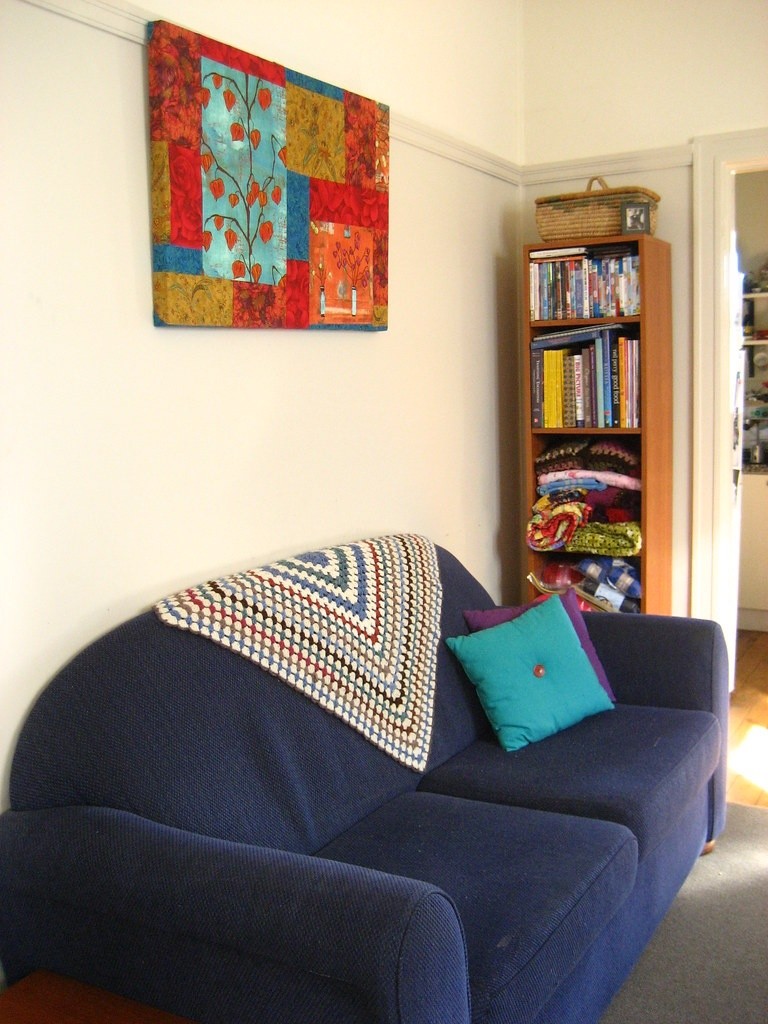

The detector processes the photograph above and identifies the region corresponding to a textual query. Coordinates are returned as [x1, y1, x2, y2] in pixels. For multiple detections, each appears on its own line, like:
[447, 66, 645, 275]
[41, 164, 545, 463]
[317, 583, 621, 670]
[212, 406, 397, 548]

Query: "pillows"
[445, 593, 615, 753]
[463, 586, 618, 702]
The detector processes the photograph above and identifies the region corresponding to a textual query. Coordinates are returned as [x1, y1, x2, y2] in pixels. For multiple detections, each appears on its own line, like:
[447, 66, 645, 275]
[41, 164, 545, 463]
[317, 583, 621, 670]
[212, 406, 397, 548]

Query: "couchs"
[0, 535, 732, 1024]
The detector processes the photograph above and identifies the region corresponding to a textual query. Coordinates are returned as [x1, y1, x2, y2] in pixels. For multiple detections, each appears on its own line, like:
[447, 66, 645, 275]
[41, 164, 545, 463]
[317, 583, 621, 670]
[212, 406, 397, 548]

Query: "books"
[529, 248, 641, 322]
[531, 324, 640, 429]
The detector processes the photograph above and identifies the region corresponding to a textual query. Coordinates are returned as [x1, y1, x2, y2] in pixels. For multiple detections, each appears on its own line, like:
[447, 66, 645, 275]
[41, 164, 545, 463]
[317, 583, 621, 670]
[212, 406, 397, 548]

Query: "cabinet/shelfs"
[736, 291, 768, 631]
[521, 231, 674, 618]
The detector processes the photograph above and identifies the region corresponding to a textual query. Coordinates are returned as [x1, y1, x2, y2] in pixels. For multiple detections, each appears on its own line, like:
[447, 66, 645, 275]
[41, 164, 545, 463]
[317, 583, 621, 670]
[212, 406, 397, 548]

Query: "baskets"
[534, 176, 662, 242]
